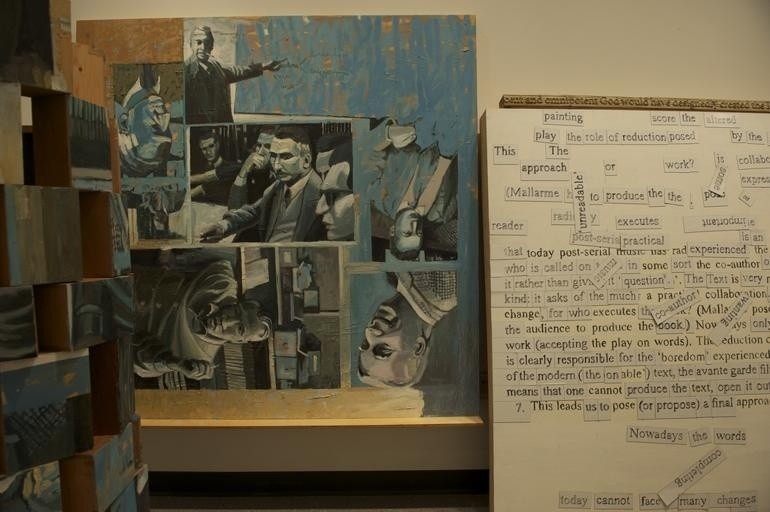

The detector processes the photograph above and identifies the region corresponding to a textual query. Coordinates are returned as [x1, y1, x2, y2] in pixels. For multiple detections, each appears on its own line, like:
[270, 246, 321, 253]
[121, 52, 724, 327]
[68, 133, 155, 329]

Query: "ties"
[408, 144, 440, 211]
[208, 162, 215, 172]
[284, 187, 292, 208]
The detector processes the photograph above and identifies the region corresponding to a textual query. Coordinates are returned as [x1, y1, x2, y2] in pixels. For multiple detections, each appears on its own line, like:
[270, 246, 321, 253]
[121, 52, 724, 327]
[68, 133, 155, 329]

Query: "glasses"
[318, 188, 353, 207]
